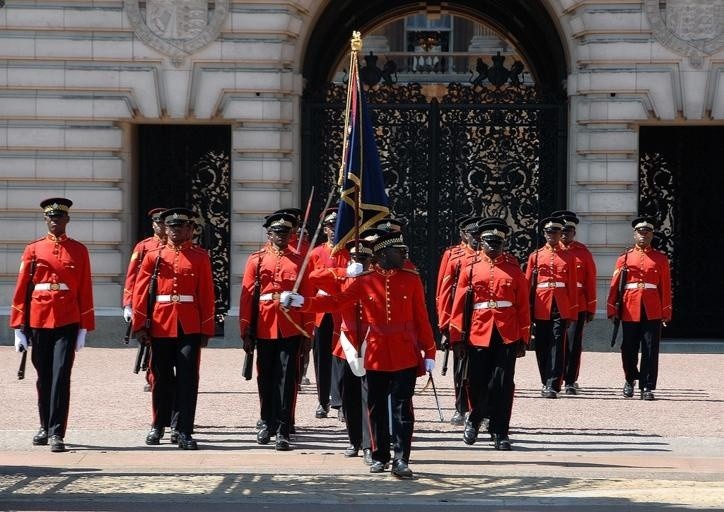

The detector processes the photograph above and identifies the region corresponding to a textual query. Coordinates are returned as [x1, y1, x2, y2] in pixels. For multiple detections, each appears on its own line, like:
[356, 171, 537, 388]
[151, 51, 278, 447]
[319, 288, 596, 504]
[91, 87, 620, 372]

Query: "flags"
[326, 50, 390, 261]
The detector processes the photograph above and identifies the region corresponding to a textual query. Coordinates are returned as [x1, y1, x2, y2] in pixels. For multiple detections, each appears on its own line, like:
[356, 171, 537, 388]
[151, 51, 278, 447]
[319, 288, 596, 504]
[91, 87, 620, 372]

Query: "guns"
[442, 258, 461, 375]
[124, 245, 159, 375]
[18, 257, 36, 380]
[529, 251, 538, 351]
[242, 260, 259, 381]
[455, 264, 474, 387]
[611, 249, 628, 346]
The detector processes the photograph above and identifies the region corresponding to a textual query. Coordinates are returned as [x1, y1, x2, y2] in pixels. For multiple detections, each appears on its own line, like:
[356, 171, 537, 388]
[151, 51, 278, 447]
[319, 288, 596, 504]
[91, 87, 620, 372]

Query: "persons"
[411, 33, 426, 72]
[425, 30, 444, 69]
[525, 209, 596, 399]
[239, 207, 436, 476]
[9, 197, 96, 453]
[606, 216, 672, 401]
[123, 208, 216, 449]
[435, 215, 532, 449]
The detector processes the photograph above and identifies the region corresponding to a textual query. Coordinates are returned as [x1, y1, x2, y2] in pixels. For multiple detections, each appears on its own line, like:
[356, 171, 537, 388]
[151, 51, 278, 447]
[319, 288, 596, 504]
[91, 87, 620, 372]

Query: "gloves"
[75, 328, 88, 352]
[346, 262, 364, 278]
[14, 328, 28, 353]
[279, 291, 304, 309]
[123, 304, 134, 323]
[424, 358, 437, 372]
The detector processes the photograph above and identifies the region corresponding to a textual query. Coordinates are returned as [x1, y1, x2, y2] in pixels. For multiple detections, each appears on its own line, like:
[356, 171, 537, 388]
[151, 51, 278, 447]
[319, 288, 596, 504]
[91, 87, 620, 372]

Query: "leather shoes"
[450, 410, 466, 425]
[344, 446, 415, 478]
[170, 428, 198, 451]
[492, 433, 511, 451]
[256, 424, 272, 445]
[274, 424, 292, 451]
[145, 424, 165, 445]
[622, 380, 637, 398]
[463, 414, 479, 446]
[50, 434, 66, 453]
[541, 383, 577, 399]
[314, 402, 330, 419]
[255, 417, 269, 426]
[32, 427, 48, 446]
[336, 406, 347, 423]
[639, 387, 656, 401]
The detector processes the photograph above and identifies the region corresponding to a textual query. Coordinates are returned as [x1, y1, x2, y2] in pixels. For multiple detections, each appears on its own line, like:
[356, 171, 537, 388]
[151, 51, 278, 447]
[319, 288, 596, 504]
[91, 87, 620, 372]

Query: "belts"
[472, 299, 512, 309]
[35, 282, 70, 292]
[257, 291, 281, 301]
[624, 282, 658, 290]
[536, 282, 567, 288]
[155, 293, 195, 303]
[576, 282, 582, 288]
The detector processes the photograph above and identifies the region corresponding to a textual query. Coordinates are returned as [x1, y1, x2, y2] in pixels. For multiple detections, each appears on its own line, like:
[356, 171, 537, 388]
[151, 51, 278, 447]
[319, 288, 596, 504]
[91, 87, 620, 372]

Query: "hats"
[631, 216, 657, 232]
[261, 206, 409, 258]
[147, 207, 201, 227]
[454, 209, 580, 245]
[39, 197, 74, 217]
